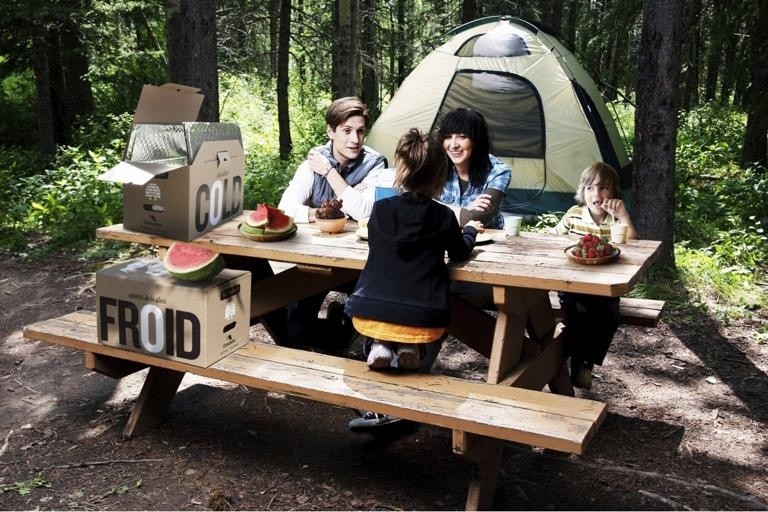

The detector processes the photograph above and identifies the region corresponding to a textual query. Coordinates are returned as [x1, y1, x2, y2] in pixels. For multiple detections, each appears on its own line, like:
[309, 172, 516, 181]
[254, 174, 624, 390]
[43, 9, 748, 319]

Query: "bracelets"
[322, 164, 333, 181]
[616, 210, 627, 219]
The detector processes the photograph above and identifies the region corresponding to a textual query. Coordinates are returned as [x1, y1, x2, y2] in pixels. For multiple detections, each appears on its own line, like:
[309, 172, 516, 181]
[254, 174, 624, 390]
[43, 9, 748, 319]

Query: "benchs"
[543, 289, 665, 328]
[25, 307, 606, 459]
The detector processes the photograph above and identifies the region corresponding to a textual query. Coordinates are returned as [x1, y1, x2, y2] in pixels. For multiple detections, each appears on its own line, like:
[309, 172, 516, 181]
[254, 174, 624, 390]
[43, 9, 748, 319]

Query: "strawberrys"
[572, 234, 612, 261]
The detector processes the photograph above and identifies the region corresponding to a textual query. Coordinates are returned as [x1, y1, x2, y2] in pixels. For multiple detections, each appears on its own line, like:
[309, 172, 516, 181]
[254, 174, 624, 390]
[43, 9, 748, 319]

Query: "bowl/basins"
[314, 215, 349, 233]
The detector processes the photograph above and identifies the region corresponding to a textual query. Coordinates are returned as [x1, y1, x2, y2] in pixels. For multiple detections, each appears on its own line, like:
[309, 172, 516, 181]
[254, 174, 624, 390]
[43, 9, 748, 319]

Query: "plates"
[240, 223, 298, 242]
[564, 243, 622, 264]
[474, 231, 494, 244]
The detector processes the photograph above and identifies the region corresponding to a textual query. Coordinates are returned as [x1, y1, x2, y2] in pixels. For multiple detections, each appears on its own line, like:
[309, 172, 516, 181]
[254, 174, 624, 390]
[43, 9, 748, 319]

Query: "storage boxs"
[97, 258, 253, 367]
[96, 79, 245, 242]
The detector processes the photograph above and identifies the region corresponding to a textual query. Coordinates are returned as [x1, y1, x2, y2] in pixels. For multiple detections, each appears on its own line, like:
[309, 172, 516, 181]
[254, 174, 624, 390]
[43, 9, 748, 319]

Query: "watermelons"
[240, 202, 292, 238]
[163, 240, 224, 283]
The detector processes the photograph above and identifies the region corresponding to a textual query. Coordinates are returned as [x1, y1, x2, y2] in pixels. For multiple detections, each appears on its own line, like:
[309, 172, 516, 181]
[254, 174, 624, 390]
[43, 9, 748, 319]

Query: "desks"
[99, 210, 662, 296]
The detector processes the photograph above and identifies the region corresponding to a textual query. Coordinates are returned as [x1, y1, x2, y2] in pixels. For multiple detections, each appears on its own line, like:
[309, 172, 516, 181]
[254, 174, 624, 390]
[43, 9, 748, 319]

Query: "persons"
[348, 107, 512, 431]
[341, 126, 486, 370]
[276, 94, 389, 355]
[536, 161, 639, 391]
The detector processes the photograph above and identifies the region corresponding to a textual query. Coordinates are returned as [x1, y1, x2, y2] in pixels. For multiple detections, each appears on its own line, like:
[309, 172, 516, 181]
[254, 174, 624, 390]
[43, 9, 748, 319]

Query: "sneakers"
[346, 411, 402, 432]
[396, 345, 421, 374]
[364, 341, 394, 371]
[571, 356, 594, 389]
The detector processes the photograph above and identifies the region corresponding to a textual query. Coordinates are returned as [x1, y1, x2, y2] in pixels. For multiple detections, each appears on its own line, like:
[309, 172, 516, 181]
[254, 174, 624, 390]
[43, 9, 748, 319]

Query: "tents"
[364, 14, 633, 223]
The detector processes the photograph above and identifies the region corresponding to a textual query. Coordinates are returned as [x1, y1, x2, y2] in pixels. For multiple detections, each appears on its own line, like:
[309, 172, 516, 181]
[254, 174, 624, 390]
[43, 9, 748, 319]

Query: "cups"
[610, 222, 628, 245]
[503, 216, 521, 237]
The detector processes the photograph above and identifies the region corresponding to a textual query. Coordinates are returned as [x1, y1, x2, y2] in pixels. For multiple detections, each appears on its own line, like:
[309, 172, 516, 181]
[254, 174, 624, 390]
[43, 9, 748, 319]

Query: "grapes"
[315, 198, 345, 220]
